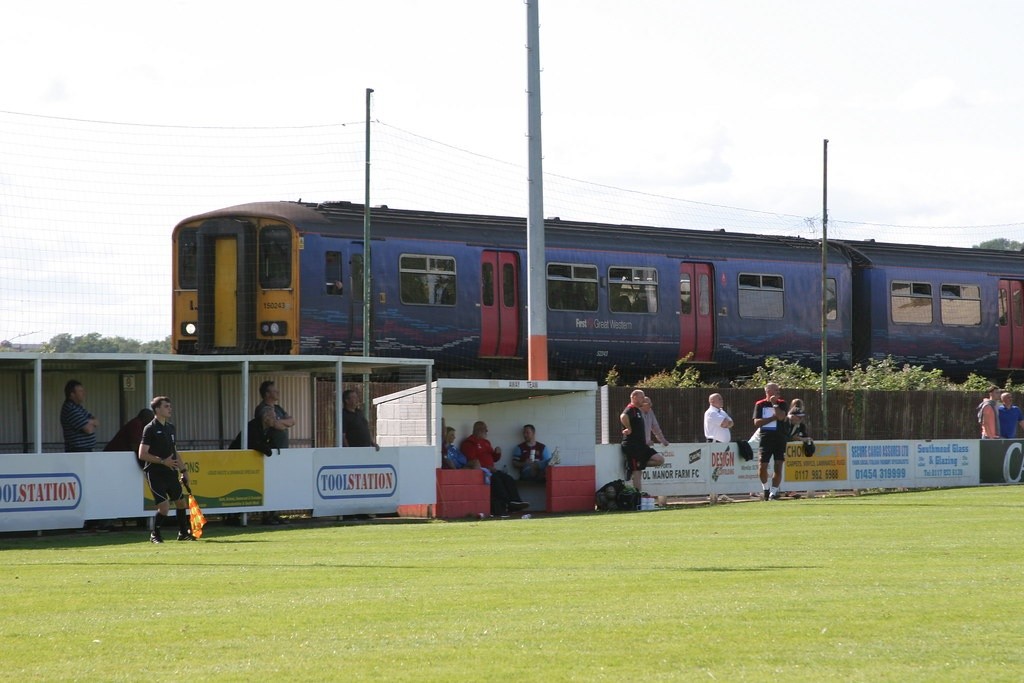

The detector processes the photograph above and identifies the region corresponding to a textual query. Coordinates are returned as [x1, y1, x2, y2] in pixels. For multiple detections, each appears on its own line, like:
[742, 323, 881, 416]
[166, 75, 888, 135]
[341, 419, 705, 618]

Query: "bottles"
[640, 495, 656, 511]
[522, 514, 533, 520]
[529, 447, 536, 462]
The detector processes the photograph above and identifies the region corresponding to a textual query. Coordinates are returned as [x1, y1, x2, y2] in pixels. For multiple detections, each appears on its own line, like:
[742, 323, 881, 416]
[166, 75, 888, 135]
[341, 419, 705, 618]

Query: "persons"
[442, 417, 467, 469]
[976, 385, 1000, 439]
[788, 399, 812, 445]
[748, 407, 806, 497]
[138, 395, 196, 544]
[703, 393, 734, 442]
[752, 383, 789, 501]
[512, 425, 561, 480]
[342, 390, 380, 451]
[255, 381, 295, 448]
[461, 421, 530, 517]
[998, 392, 1024, 439]
[620, 390, 665, 493]
[639, 397, 668, 448]
[60, 379, 109, 533]
[226, 401, 276, 456]
[103, 408, 154, 451]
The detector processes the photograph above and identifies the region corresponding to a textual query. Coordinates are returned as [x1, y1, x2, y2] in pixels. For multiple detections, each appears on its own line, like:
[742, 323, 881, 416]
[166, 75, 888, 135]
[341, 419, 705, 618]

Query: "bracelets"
[181, 469, 186, 473]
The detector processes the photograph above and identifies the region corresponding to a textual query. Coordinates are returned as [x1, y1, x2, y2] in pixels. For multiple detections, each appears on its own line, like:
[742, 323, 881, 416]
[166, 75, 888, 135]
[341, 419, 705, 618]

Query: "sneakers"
[150, 531, 163, 544]
[177, 531, 196, 541]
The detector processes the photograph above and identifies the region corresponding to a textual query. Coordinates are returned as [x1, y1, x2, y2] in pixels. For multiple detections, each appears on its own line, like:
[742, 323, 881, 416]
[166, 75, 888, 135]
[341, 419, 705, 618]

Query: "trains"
[171, 198, 1024, 389]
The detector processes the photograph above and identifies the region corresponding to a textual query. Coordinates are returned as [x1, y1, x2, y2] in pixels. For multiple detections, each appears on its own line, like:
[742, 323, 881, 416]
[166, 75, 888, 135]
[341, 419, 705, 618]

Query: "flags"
[189, 493, 207, 539]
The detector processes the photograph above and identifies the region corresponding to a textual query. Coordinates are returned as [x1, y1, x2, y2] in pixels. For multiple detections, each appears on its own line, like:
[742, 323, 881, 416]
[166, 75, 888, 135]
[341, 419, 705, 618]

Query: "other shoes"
[264, 519, 279, 524]
[365, 516, 374, 520]
[346, 516, 358, 521]
[510, 500, 529, 508]
[95, 526, 109, 532]
[76, 526, 88, 532]
[763, 488, 770, 501]
[625, 459, 633, 481]
[279, 518, 290, 524]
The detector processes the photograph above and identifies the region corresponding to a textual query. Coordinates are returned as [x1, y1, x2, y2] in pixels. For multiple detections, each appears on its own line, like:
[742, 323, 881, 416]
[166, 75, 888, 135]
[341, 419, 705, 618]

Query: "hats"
[789, 407, 805, 417]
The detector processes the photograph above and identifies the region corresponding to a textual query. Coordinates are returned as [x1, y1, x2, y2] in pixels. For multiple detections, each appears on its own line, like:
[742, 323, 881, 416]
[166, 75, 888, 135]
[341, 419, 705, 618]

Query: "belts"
[707, 439, 720, 442]
